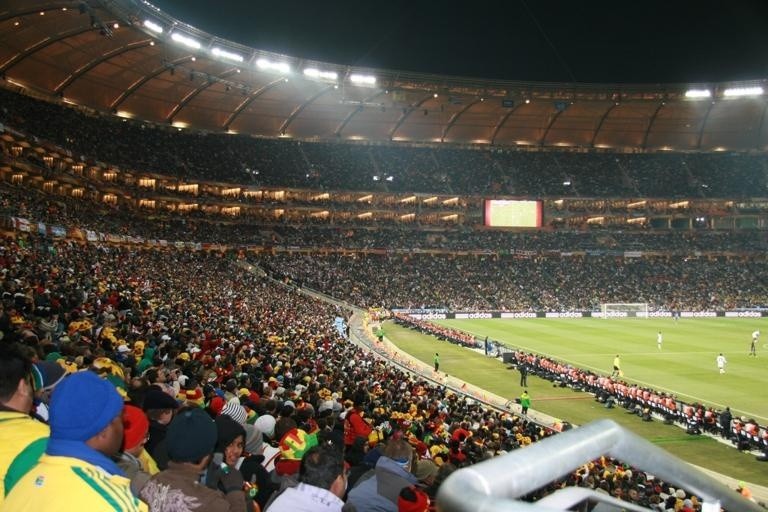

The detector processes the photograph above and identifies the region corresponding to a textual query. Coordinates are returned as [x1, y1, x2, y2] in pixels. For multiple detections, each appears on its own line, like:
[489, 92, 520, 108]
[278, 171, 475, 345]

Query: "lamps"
[210, 45, 246, 65]
[142, 19, 164, 34]
[252, 56, 293, 78]
[348, 72, 379, 86]
[721, 84, 766, 102]
[681, 86, 714, 102]
[302, 67, 341, 84]
[169, 31, 202, 51]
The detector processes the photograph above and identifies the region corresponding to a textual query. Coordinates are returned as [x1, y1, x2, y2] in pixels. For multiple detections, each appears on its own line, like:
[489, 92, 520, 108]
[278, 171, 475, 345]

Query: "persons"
[0, 86, 768, 509]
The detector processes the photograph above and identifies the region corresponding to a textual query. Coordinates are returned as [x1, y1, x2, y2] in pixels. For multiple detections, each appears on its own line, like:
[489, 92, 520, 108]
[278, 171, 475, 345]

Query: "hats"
[121, 404, 149, 449]
[167, 408, 216, 461]
[145, 391, 178, 410]
[210, 397, 318, 459]
[398, 460, 439, 512]
[48, 372, 121, 442]
